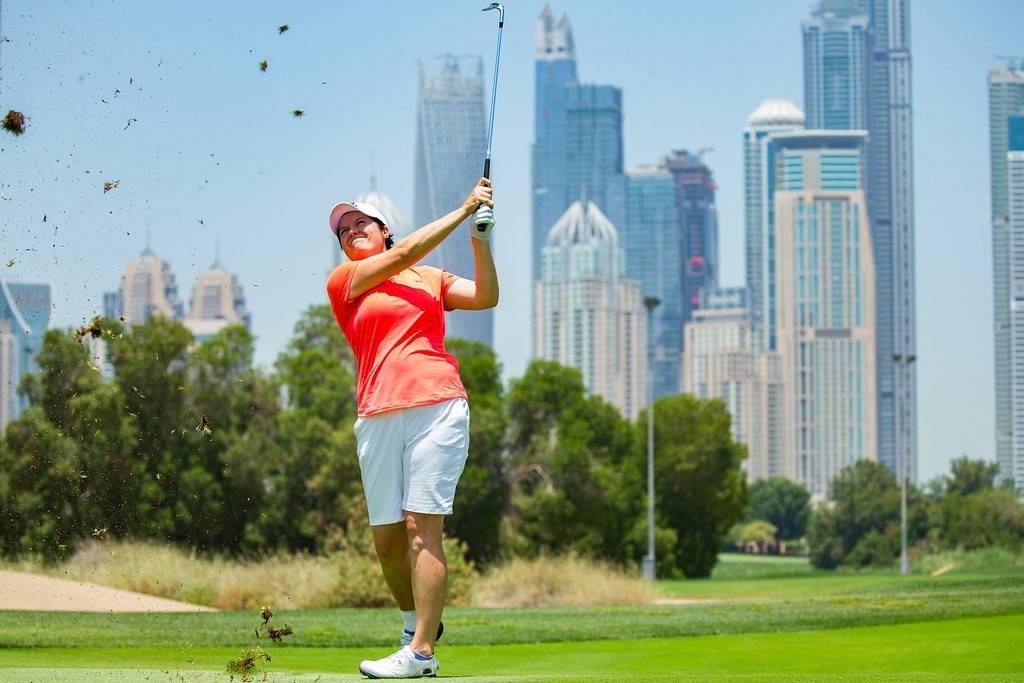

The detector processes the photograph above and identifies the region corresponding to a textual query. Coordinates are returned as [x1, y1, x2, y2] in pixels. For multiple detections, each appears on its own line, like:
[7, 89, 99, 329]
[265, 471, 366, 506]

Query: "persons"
[327, 178, 499, 678]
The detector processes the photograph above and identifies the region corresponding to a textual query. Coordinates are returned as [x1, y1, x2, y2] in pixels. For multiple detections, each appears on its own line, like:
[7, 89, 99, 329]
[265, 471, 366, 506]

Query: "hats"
[330, 202, 388, 235]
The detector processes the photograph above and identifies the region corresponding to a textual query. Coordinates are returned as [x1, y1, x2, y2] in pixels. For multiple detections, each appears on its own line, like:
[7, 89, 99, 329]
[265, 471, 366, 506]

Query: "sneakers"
[359, 645, 436, 679]
[400, 622, 444, 651]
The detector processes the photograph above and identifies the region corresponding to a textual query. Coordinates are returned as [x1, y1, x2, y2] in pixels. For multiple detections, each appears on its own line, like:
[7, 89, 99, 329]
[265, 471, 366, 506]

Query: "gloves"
[470, 204, 495, 241]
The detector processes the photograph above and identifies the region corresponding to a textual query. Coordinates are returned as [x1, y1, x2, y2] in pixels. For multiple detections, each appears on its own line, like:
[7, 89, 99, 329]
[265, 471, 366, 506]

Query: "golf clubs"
[476, 2, 505, 233]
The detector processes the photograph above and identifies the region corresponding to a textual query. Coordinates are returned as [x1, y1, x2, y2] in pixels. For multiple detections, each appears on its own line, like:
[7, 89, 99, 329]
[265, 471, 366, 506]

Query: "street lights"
[890, 348, 919, 570]
[634, 292, 664, 584]
